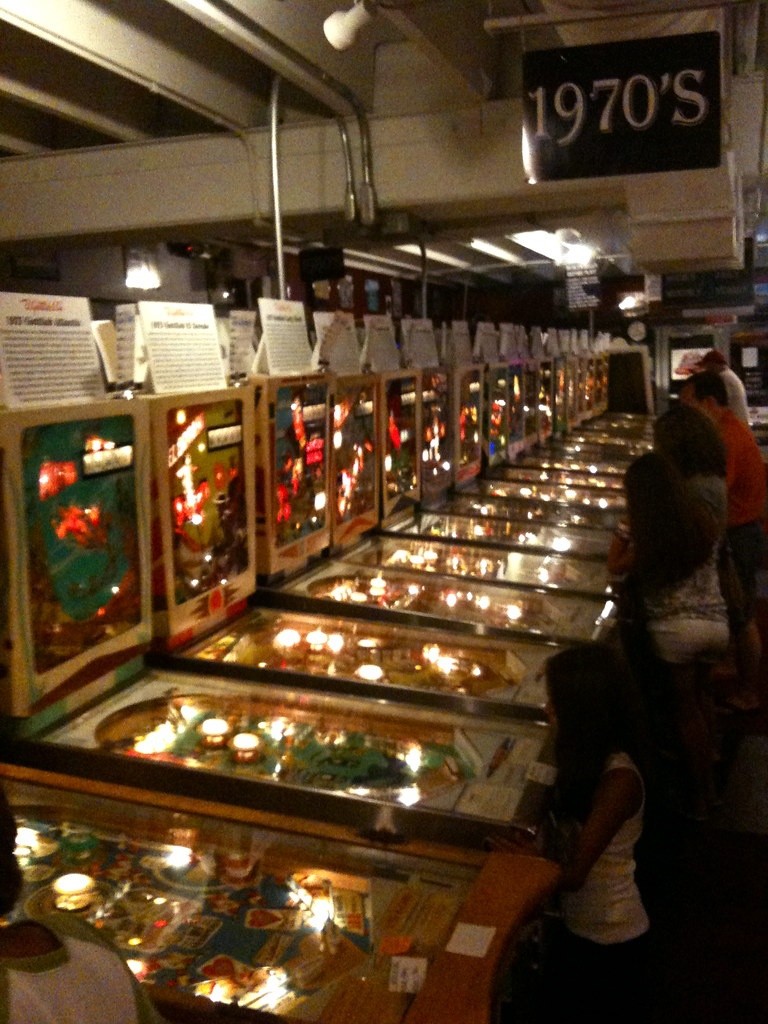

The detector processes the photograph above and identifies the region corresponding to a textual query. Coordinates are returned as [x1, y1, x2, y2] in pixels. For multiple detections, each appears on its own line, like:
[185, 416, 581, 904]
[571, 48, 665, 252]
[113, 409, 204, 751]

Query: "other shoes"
[726, 690, 762, 711]
[676, 791, 720, 819]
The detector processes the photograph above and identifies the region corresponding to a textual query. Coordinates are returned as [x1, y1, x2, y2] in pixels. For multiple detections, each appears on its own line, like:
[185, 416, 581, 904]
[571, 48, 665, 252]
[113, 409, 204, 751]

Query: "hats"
[695, 351, 727, 368]
[674, 353, 705, 375]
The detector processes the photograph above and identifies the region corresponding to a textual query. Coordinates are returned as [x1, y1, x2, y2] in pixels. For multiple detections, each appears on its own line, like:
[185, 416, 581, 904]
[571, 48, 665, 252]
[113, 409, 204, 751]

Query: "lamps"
[323, 0, 372, 52]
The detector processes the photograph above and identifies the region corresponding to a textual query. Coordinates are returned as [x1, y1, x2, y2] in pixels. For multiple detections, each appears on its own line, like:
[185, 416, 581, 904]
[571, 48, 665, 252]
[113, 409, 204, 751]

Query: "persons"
[1, 787, 166, 1023]
[497, 349, 768, 1024]
